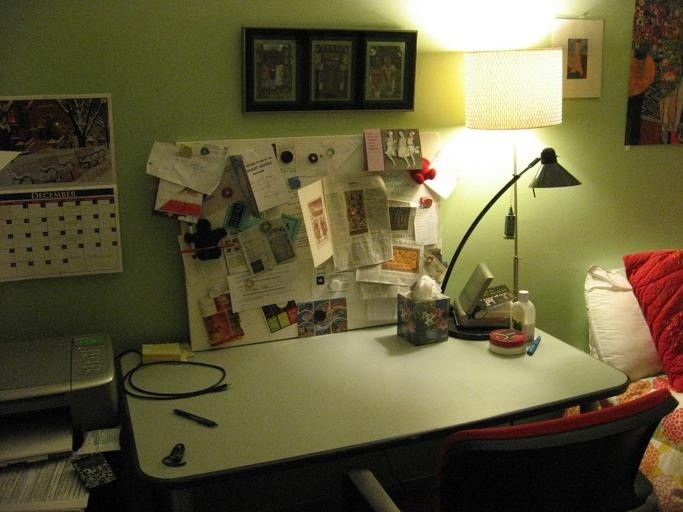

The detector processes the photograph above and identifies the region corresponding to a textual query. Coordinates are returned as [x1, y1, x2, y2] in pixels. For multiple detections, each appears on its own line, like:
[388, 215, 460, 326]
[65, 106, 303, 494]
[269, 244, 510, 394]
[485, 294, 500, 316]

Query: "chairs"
[334, 387, 676, 511]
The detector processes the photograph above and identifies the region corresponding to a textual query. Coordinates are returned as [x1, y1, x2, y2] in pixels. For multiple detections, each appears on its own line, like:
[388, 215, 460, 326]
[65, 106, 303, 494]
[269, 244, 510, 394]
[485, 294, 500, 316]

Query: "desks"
[117, 321, 636, 509]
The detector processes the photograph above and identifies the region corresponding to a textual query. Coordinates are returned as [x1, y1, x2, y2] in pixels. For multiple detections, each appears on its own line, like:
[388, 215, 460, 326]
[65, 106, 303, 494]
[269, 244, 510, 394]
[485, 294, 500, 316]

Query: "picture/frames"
[553, 14, 603, 100]
[239, 24, 417, 114]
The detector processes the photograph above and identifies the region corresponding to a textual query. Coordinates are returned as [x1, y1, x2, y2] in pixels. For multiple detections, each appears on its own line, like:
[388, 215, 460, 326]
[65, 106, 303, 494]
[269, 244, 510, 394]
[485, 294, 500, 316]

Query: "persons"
[569, 40, 584, 76]
[369, 53, 396, 99]
[260, 58, 286, 98]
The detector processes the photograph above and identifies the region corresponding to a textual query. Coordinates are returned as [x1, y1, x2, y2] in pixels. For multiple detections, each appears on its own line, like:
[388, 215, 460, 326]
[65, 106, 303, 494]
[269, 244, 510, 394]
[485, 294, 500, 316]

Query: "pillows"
[621, 244, 683, 393]
[582, 263, 662, 385]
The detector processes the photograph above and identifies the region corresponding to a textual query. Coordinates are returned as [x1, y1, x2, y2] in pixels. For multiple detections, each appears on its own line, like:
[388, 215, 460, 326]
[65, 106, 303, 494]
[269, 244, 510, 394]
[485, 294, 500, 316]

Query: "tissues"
[396, 275, 450, 346]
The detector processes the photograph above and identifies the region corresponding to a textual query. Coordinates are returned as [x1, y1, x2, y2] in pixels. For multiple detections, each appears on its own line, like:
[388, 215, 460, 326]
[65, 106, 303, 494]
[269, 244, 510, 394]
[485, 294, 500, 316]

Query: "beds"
[561, 360, 683, 512]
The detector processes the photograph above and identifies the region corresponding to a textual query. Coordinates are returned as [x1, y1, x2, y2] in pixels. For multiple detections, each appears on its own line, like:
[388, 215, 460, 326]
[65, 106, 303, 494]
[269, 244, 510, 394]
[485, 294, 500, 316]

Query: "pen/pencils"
[526, 336, 541, 356]
[173, 408, 219, 428]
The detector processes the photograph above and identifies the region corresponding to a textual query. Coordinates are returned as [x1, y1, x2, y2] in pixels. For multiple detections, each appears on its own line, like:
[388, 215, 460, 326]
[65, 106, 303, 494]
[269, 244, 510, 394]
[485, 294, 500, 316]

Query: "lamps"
[460, 46, 572, 304]
[441, 145, 582, 296]
